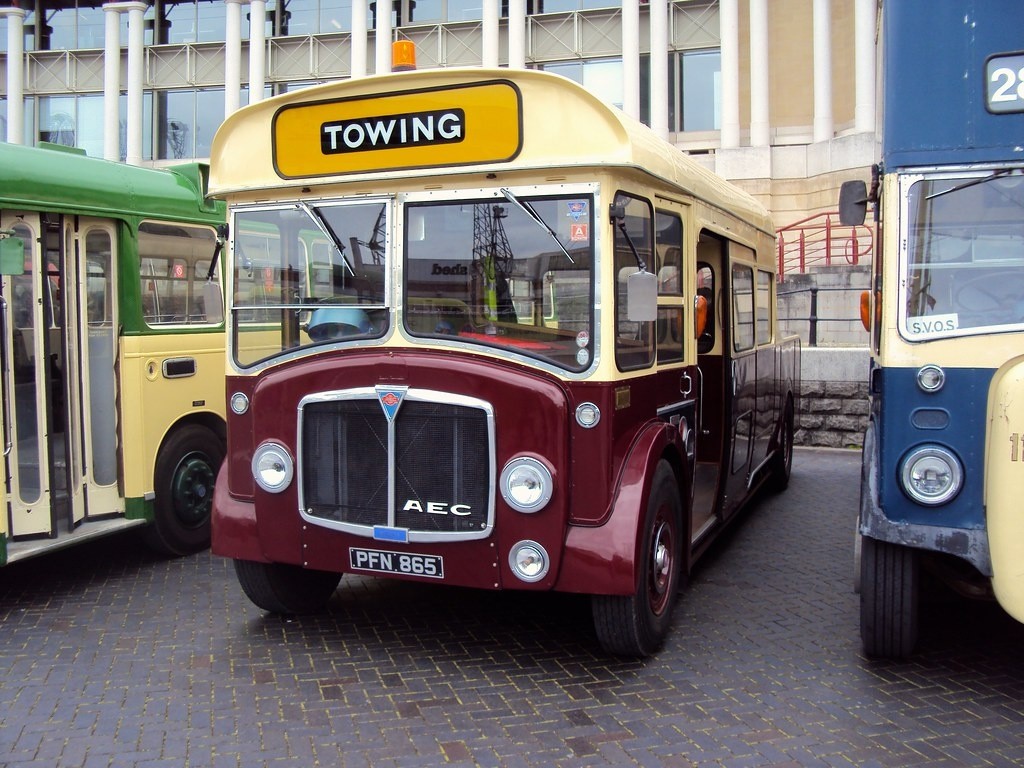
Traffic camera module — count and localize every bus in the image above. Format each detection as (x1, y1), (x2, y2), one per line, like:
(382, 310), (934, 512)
(0, 140), (561, 570)
(203, 67), (800, 660)
(841, 0), (1024, 669)
